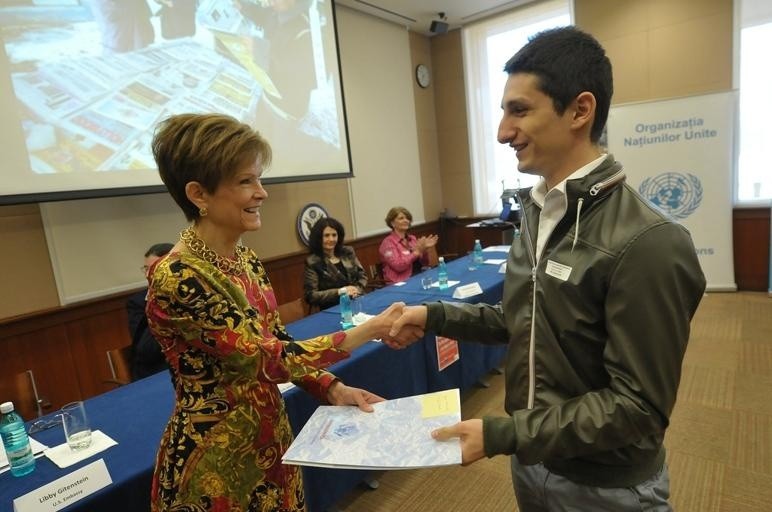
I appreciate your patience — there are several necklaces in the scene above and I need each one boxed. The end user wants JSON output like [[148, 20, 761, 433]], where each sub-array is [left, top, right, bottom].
[[178, 227, 248, 276]]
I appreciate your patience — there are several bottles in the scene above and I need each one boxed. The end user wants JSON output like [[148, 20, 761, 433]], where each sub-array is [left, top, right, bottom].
[[337, 287, 354, 330], [473, 239, 483, 264], [438, 256, 449, 291], [0, 401, 37, 477]]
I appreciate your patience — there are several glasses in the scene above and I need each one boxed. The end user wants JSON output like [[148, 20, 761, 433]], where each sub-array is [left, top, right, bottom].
[[28, 412, 70, 436]]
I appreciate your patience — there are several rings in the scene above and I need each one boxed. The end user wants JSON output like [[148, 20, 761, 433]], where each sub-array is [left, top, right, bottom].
[[430, 239, 433, 240]]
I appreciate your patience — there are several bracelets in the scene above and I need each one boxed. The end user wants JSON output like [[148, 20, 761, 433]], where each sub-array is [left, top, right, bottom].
[[339, 288, 346, 295]]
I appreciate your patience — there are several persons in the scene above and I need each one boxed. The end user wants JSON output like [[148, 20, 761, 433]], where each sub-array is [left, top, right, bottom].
[[143, 110, 428, 511], [83, 0, 321, 117], [303, 217, 370, 312], [124, 242, 174, 383], [376, 22, 709, 510], [377, 206, 440, 286]]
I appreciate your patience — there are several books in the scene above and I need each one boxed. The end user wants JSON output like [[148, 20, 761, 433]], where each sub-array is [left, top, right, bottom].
[[281, 387, 464, 471]]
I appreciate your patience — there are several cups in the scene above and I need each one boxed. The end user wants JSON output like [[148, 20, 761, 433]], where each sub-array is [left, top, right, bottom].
[[421, 266, 433, 290], [60, 400, 92, 453], [352, 294, 364, 314], [467, 251, 477, 270]]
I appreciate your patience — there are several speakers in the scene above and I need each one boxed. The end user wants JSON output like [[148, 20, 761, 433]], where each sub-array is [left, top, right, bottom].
[[431, 21, 450, 34]]
